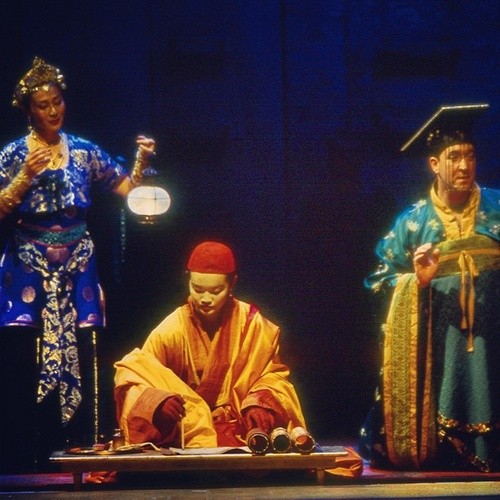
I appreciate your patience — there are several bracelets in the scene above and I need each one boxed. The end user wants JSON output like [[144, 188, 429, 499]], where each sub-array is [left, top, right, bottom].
[[0, 170, 34, 217], [128, 151, 150, 190]]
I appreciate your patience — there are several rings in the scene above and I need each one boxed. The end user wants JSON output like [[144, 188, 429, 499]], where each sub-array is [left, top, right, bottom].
[[38, 160, 41, 164]]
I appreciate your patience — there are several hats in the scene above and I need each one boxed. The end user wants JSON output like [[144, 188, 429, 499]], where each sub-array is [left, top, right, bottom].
[[187, 242, 237, 274], [400, 103, 490, 193], [12, 56, 66, 114]]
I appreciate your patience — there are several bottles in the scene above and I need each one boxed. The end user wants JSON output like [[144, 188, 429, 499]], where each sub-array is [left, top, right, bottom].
[[113, 429, 125, 452]]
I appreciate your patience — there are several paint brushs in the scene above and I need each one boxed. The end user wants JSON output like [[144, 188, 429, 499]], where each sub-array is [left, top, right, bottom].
[[181, 415, 185, 451]]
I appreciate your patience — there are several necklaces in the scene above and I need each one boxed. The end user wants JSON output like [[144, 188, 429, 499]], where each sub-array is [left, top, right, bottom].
[[31, 127, 61, 167]]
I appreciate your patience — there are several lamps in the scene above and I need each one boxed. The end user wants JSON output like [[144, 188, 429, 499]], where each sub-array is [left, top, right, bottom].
[[127, 140, 172, 223]]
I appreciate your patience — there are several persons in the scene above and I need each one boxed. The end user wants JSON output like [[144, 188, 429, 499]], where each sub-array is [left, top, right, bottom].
[[85, 241, 363, 484], [0, 56, 156, 472], [363, 104, 500, 473]]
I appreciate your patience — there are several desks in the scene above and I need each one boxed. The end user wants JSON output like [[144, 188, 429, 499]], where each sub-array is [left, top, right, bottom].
[[48, 445, 349, 489]]
